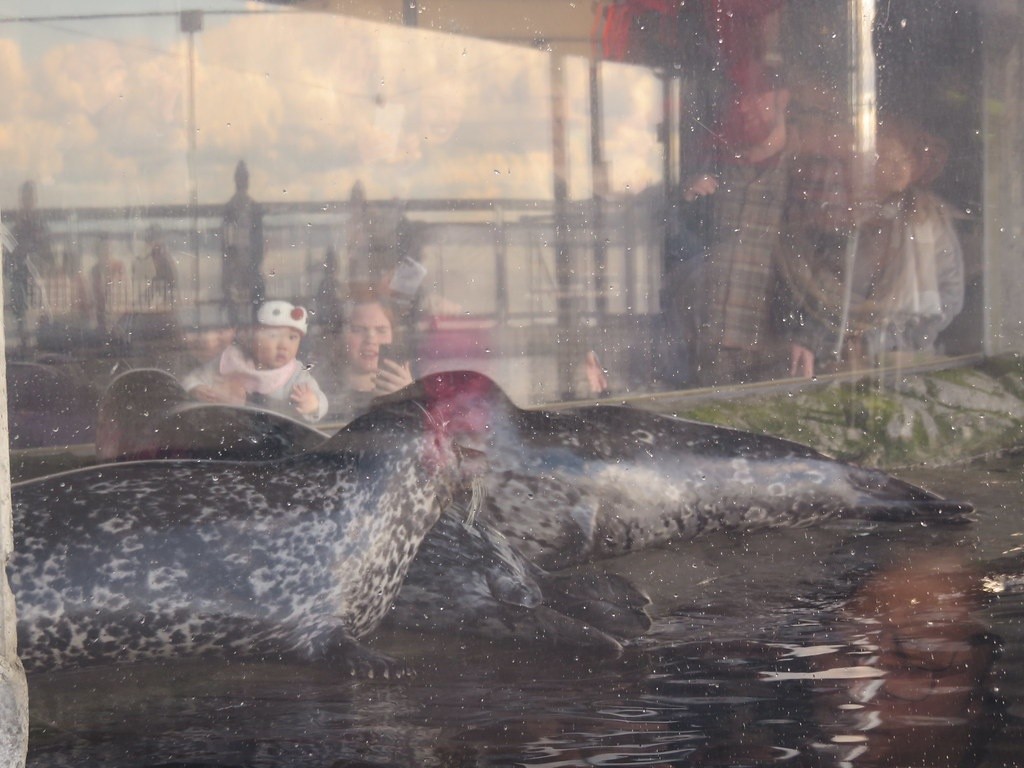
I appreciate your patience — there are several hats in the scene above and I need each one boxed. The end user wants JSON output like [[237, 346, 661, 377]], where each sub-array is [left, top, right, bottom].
[[234, 293, 308, 336]]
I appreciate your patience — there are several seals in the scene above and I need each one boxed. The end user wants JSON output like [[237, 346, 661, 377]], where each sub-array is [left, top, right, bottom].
[[102, 407, 543, 629], [532, 406, 976, 558], [4, 371, 581, 671]]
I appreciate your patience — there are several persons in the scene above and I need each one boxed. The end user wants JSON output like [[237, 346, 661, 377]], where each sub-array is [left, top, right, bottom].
[[672, 59, 965, 385], [180, 297, 328, 427], [8, 176, 431, 341], [303, 285, 415, 422]]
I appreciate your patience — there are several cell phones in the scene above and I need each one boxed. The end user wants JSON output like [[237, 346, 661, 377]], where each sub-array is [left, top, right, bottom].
[[377, 344, 407, 371]]
